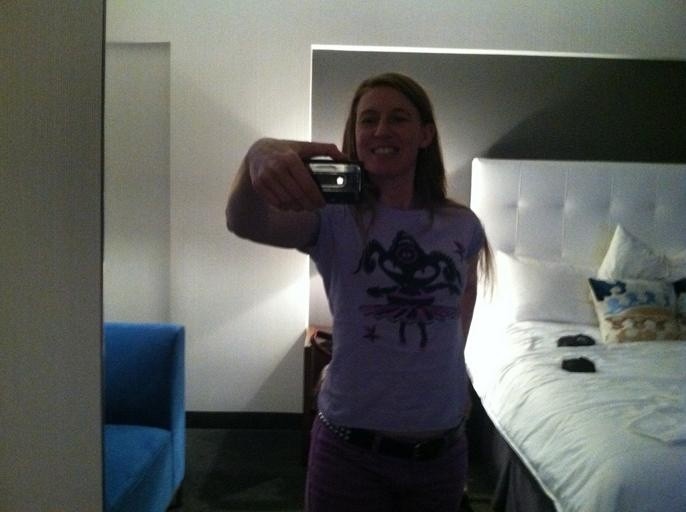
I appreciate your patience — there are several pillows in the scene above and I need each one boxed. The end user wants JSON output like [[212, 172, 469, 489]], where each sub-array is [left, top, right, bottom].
[[498, 251, 598, 328], [589, 222, 686, 299], [604, 306, 685, 342], [590, 279, 685, 321]]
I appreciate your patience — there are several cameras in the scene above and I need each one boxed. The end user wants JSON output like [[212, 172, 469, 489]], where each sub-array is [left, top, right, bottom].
[[306, 158, 365, 204]]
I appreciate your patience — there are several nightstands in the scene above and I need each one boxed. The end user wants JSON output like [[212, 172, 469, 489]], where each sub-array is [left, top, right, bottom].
[[303, 323, 336, 421]]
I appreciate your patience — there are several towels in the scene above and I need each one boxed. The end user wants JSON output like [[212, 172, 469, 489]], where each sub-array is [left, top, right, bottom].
[[627, 407, 685, 442]]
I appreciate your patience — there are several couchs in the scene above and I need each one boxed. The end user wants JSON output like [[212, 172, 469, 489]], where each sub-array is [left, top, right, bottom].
[[102, 324, 187, 507]]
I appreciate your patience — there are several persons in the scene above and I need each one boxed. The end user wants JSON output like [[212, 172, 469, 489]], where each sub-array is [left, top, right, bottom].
[[225, 72, 499, 511]]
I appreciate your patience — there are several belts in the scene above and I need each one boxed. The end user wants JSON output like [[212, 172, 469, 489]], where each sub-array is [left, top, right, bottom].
[[317, 406, 469, 462]]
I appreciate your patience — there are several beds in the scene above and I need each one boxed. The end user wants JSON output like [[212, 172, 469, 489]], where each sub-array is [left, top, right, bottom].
[[463, 158, 685, 507]]
